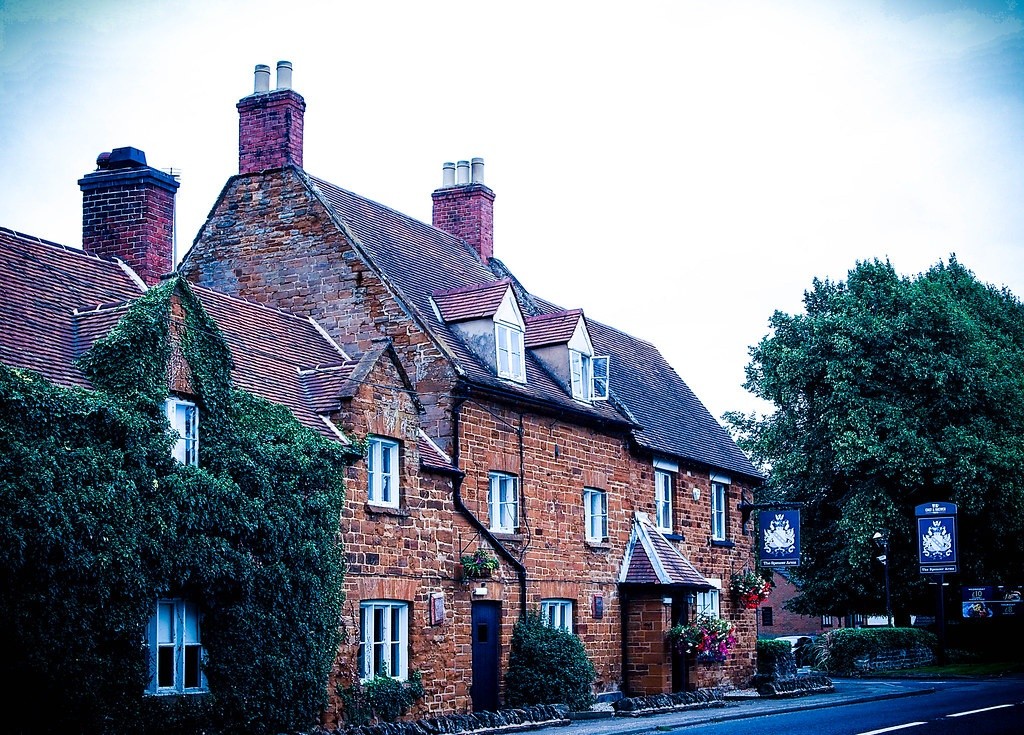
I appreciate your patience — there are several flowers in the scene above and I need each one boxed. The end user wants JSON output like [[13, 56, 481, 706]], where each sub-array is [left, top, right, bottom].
[[462, 548, 500, 577], [729, 569, 772, 609], [665, 615, 737, 663]]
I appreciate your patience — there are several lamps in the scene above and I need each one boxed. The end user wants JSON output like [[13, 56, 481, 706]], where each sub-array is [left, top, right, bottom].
[[687, 592, 696, 606]]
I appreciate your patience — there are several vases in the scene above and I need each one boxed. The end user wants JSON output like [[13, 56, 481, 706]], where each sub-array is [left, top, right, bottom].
[[672, 642, 688, 655], [468, 568, 492, 578]]
